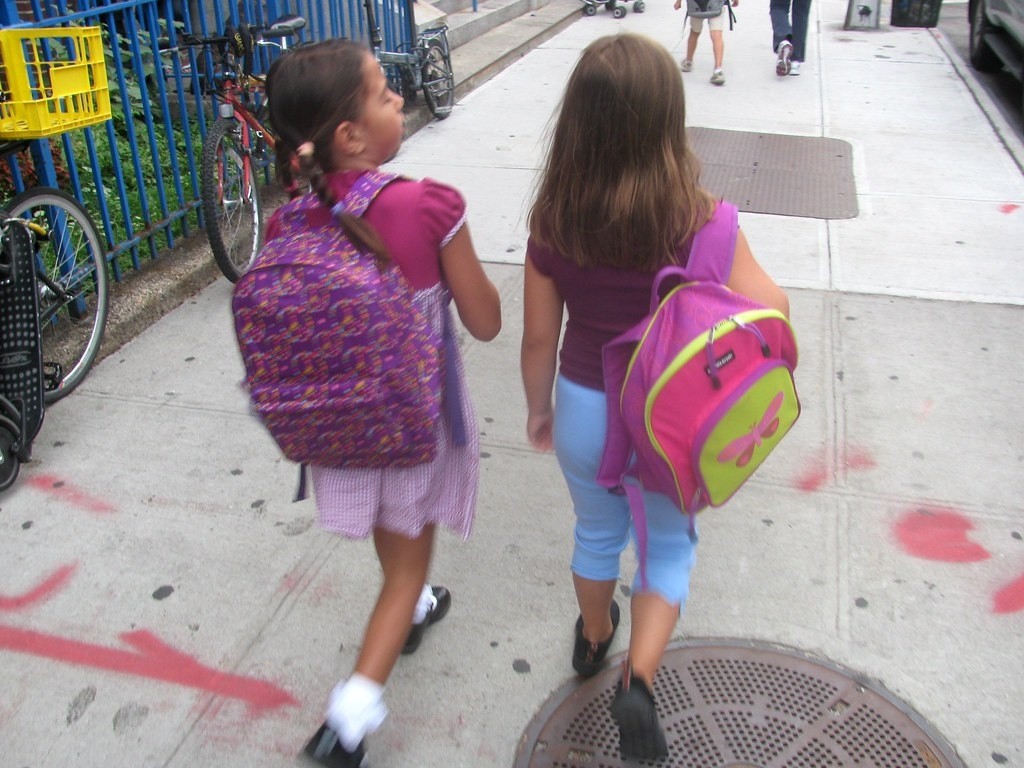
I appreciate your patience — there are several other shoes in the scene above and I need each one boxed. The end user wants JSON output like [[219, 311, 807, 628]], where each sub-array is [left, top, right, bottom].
[[572, 597, 620, 676], [402, 585, 451, 656], [295, 712, 368, 768], [608, 658, 670, 759]]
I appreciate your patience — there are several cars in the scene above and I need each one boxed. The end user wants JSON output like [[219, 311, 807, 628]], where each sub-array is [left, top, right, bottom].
[[965, 0, 1024, 90]]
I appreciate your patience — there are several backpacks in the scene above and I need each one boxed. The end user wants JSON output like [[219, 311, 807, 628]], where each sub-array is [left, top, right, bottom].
[[596, 199, 803, 588], [681, 0, 737, 31], [231, 169, 467, 503]]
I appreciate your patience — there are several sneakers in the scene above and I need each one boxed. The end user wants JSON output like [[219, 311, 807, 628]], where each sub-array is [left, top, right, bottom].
[[775, 39, 794, 77], [788, 59, 803, 75], [711, 71, 727, 85], [681, 59, 693, 73]]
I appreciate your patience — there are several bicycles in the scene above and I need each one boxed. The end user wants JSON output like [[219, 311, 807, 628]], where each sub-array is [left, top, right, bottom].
[[150, 0, 454, 283], [0, 139, 109, 488]]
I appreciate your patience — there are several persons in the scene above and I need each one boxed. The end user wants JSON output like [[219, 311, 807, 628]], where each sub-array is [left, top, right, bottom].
[[770, 0, 813, 76], [674, 0, 739, 83], [263, 38, 501, 768], [520, 35, 789, 757]]
